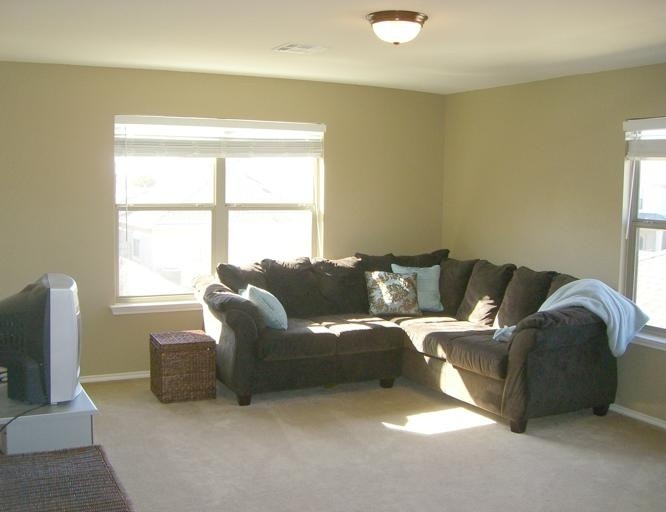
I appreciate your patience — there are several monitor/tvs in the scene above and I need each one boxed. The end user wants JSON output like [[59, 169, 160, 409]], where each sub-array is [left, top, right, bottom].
[[0, 272, 82, 406]]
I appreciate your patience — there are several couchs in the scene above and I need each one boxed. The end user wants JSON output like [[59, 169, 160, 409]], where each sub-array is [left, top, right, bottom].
[[195, 249, 618, 433]]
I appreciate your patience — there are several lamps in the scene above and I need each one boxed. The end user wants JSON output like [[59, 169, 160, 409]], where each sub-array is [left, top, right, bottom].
[[365, 11, 430, 46]]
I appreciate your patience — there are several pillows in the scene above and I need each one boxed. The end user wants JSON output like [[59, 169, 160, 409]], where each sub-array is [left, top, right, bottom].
[[234, 282, 291, 335], [361, 263, 446, 320]]
